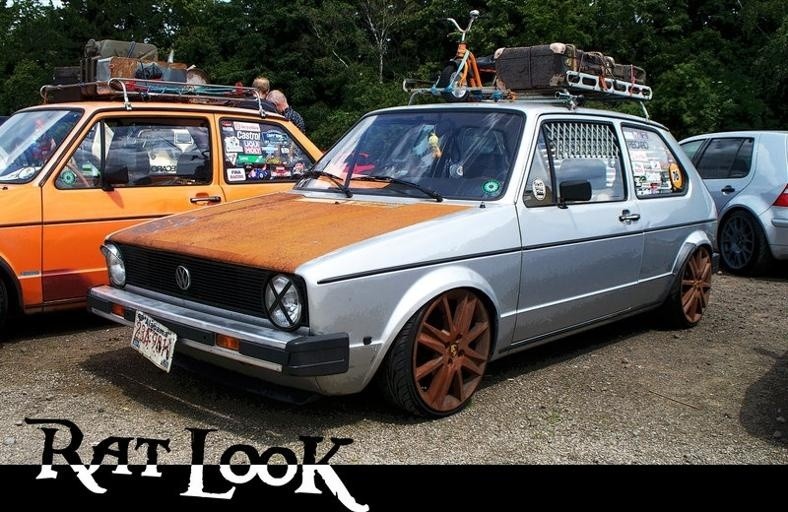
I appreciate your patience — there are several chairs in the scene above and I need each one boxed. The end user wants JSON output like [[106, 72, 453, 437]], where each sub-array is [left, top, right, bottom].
[[476, 153, 607, 191], [104, 143, 210, 184]]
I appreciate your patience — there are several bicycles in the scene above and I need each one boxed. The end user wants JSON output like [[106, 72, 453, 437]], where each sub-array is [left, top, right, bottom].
[[436, 10, 490, 102]]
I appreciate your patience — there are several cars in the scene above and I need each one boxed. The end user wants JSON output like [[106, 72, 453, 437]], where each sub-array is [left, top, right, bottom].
[[0, 70, 787, 420]]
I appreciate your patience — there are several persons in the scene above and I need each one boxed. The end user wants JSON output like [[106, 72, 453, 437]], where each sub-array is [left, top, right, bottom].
[[238, 75, 277, 112], [267, 90, 306, 133]]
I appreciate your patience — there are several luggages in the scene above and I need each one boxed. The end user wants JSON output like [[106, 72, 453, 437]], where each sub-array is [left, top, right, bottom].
[[493, 42, 649, 94], [47, 39, 187, 102]]
